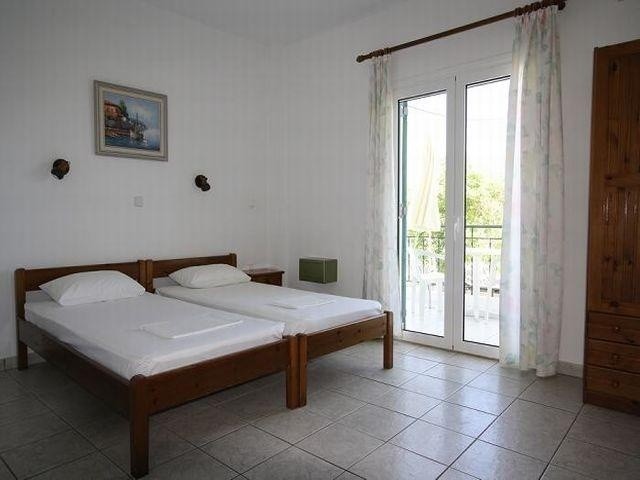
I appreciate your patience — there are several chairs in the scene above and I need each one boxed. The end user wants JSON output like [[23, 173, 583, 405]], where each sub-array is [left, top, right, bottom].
[[407, 245, 504, 323]]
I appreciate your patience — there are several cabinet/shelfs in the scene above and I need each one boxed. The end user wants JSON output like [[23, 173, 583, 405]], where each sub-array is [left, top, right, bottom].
[[580, 38, 640, 416]]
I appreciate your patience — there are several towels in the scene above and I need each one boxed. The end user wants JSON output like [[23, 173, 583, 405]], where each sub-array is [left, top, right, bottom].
[[137, 310, 244, 340], [265, 292, 337, 310]]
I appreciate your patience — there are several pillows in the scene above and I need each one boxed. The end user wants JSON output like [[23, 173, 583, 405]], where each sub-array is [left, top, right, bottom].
[[168, 263, 252, 289], [36, 269, 146, 306]]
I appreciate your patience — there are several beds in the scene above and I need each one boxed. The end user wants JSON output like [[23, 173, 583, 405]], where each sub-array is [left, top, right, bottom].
[[14, 259, 300, 480], [144, 252, 394, 408]]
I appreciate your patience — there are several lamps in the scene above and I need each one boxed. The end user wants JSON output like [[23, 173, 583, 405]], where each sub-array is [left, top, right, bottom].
[[195, 174, 210, 191], [50, 159, 70, 180]]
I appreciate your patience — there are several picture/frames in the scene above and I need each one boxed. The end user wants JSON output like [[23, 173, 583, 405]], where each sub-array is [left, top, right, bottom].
[[93, 79, 169, 162]]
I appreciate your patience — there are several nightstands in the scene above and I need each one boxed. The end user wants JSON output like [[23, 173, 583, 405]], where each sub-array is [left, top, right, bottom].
[[241, 267, 284, 286]]
